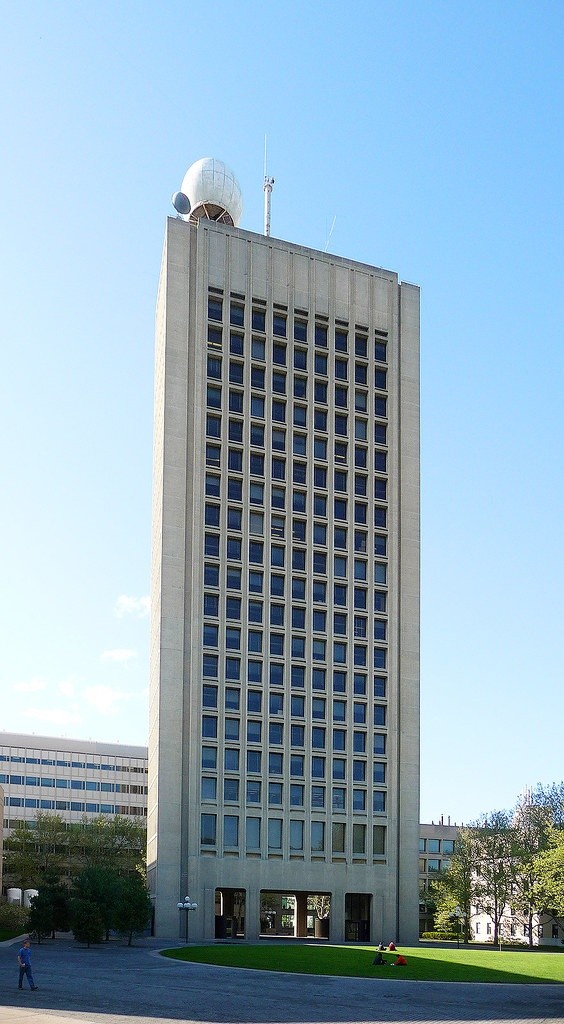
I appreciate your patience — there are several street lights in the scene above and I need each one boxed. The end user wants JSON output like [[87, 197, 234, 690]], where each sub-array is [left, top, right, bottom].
[[176, 896, 198, 943], [449, 906, 466, 949]]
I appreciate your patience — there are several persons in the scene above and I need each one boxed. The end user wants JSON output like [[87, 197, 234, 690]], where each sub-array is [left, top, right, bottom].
[[378, 941, 386, 951], [389, 941, 397, 951], [393, 954, 407, 965], [372, 953, 387, 965], [17, 940, 38, 991]]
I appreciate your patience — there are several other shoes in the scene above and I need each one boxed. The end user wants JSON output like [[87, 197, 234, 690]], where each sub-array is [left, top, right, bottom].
[[17, 987, 24, 990], [31, 987, 38, 991]]
